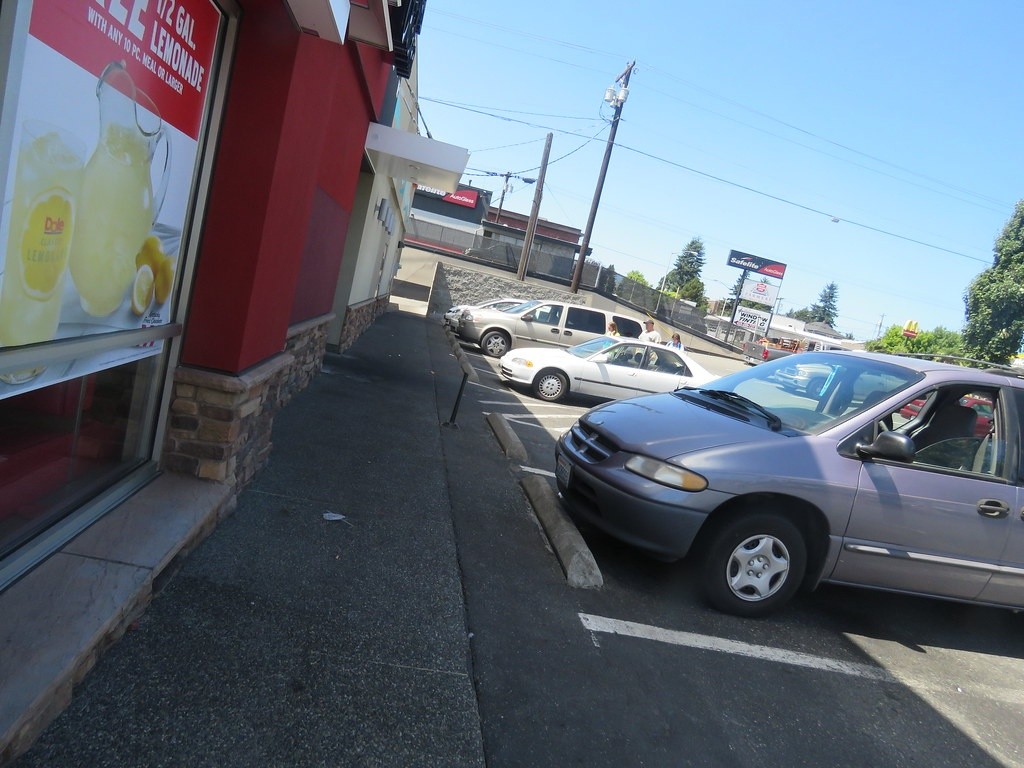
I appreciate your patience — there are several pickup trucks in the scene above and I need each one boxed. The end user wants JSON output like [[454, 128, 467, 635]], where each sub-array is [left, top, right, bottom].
[[744, 342, 793, 367]]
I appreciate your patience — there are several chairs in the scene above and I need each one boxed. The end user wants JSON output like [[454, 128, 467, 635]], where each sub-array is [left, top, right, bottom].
[[635, 353, 642, 361], [849, 391, 893, 445], [910, 406, 978, 469]]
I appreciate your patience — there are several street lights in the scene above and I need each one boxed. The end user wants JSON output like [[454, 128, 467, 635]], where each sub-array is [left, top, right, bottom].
[[712, 278, 732, 338], [654, 250, 698, 315]]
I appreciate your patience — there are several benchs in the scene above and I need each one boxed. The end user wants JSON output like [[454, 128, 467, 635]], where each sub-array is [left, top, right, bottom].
[[650, 359, 681, 375]]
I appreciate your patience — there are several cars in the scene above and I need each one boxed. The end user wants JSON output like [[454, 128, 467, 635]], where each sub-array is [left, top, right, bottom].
[[443, 299, 574, 333], [498, 335, 722, 402], [552, 350, 1024, 622], [775, 365, 886, 401]]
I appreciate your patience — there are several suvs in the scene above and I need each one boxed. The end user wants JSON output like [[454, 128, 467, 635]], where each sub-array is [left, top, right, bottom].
[[457, 298, 648, 360]]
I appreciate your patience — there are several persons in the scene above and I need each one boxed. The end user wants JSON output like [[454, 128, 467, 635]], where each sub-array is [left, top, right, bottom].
[[636, 320, 660, 364], [604, 321, 620, 352], [666, 333, 684, 351]]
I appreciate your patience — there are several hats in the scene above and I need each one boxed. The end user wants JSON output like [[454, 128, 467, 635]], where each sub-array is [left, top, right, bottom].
[[643, 320, 654, 325]]
